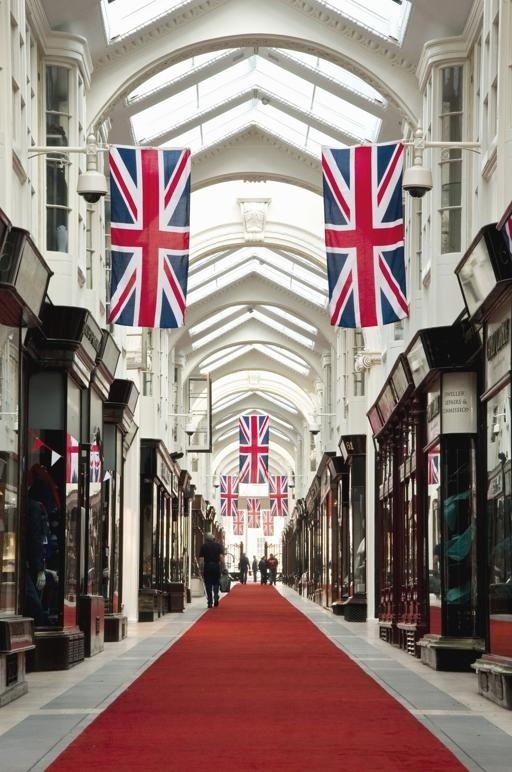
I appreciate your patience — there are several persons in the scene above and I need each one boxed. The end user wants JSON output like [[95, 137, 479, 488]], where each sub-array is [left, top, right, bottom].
[[201, 532, 225, 607], [239, 553, 251, 585], [259, 556, 271, 586], [20, 492, 59, 618], [268, 553, 278, 585], [252, 555, 258, 581]]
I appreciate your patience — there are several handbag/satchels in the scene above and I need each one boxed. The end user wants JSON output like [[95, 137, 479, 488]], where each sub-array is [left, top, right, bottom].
[[219, 575, 230, 592]]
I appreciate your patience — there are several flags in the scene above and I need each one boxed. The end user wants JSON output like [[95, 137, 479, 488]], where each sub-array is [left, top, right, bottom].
[[268, 475, 289, 516], [426, 447, 440, 485], [90, 442, 102, 482], [320, 141, 409, 328], [105, 145, 192, 330], [219, 475, 239, 517], [233, 510, 244, 534], [238, 415, 271, 482], [246, 498, 260, 527], [263, 510, 274, 536], [65, 435, 79, 483]]
